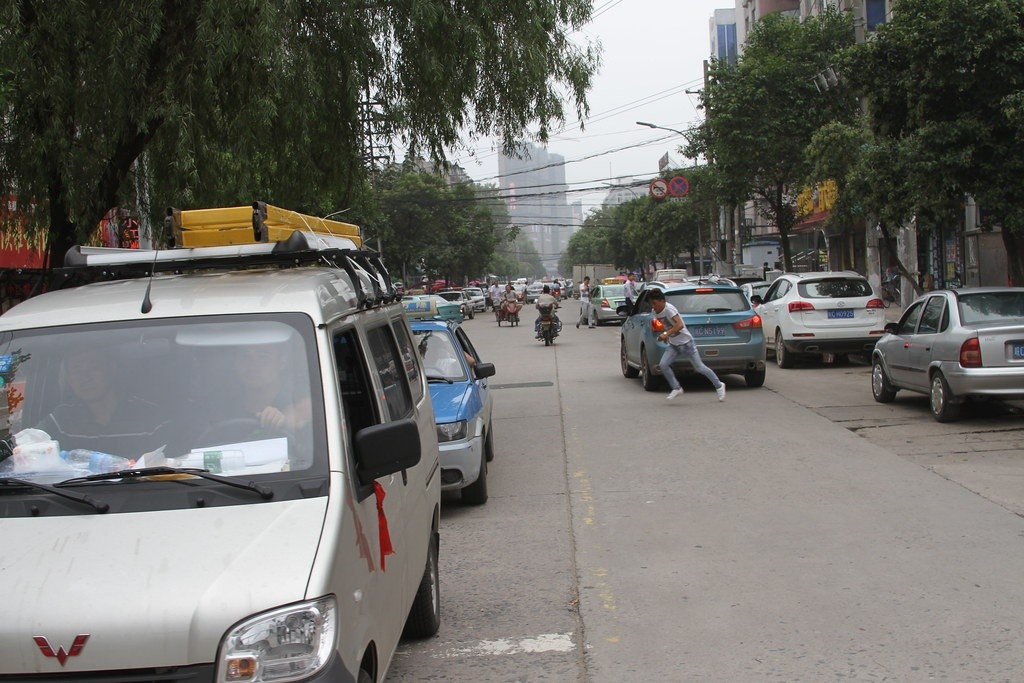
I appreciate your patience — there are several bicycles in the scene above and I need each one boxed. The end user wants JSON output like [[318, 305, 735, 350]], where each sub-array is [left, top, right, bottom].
[[877, 275, 901, 309]]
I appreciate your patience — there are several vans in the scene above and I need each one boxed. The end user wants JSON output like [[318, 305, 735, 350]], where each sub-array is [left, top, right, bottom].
[[0, 231, 443, 683]]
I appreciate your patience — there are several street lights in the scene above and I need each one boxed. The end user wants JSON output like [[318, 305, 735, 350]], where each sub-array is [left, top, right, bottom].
[[635, 121, 703, 278], [603, 183, 651, 284]]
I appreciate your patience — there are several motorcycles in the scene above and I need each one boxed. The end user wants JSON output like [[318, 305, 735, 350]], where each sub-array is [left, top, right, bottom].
[[535, 298, 562, 346]]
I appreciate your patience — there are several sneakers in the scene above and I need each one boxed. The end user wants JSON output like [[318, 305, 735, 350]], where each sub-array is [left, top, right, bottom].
[[716, 383, 726, 401], [667, 388, 683, 400]]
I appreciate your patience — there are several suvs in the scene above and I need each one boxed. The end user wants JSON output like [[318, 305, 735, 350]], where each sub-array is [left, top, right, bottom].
[[616, 278, 766, 391], [750, 270, 887, 369]]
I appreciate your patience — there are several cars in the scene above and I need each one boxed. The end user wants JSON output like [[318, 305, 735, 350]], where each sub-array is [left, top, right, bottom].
[[580, 269, 773, 326], [396, 289, 463, 325], [400, 300, 496, 507], [394, 276, 573, 320], [872, 286, 1024, 422]]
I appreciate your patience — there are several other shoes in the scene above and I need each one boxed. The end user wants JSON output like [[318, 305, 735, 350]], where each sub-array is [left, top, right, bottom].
[[589, 327, 594, 329], [576, 323, 579, 328]]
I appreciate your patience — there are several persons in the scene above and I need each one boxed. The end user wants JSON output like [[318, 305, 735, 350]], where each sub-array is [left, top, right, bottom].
[[464, 351, 475, 365], [575, 276, 595, 328], [624, 273, 635, 305], [552, 279, 560, 290], [534, 286, 557, 339], [38, 353, 162, 443], [647, 288, 726, 401], [489, 281, 523, 312], [763, 262, 770, 280], [242, 348, 312, 432]]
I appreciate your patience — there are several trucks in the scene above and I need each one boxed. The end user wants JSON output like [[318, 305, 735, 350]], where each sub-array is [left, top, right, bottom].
[[573, 264, 629, 299]]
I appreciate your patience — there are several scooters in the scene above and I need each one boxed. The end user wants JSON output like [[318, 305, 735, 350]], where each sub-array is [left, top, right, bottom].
[[497, 298, 518, 326]]
